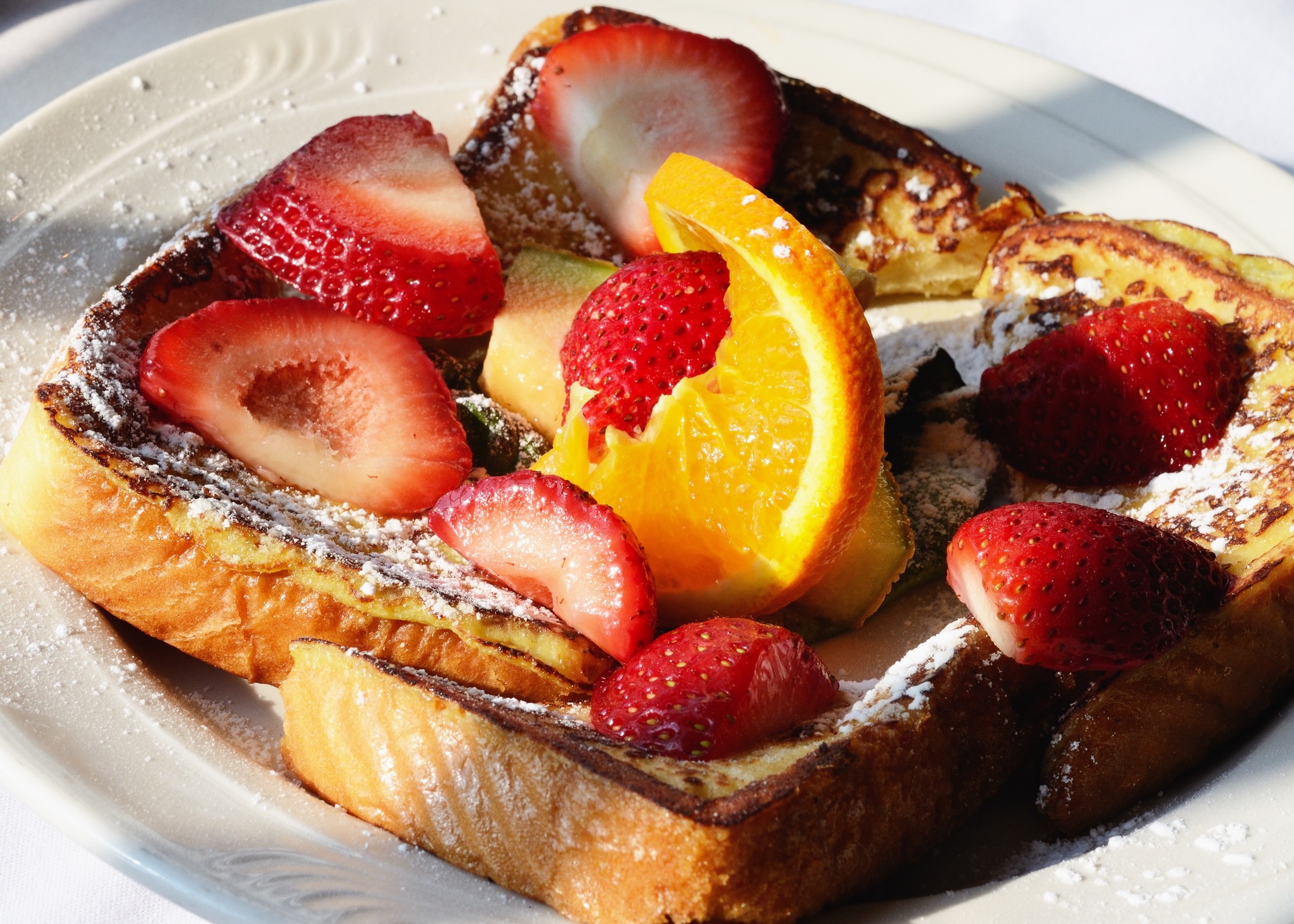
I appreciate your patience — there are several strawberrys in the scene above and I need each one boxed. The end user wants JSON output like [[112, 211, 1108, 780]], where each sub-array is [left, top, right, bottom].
[[153, 0, 1254, 767]]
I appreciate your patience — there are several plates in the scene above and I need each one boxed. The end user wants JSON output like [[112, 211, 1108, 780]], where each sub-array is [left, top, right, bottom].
[[1, 1, 1293, 924]]
